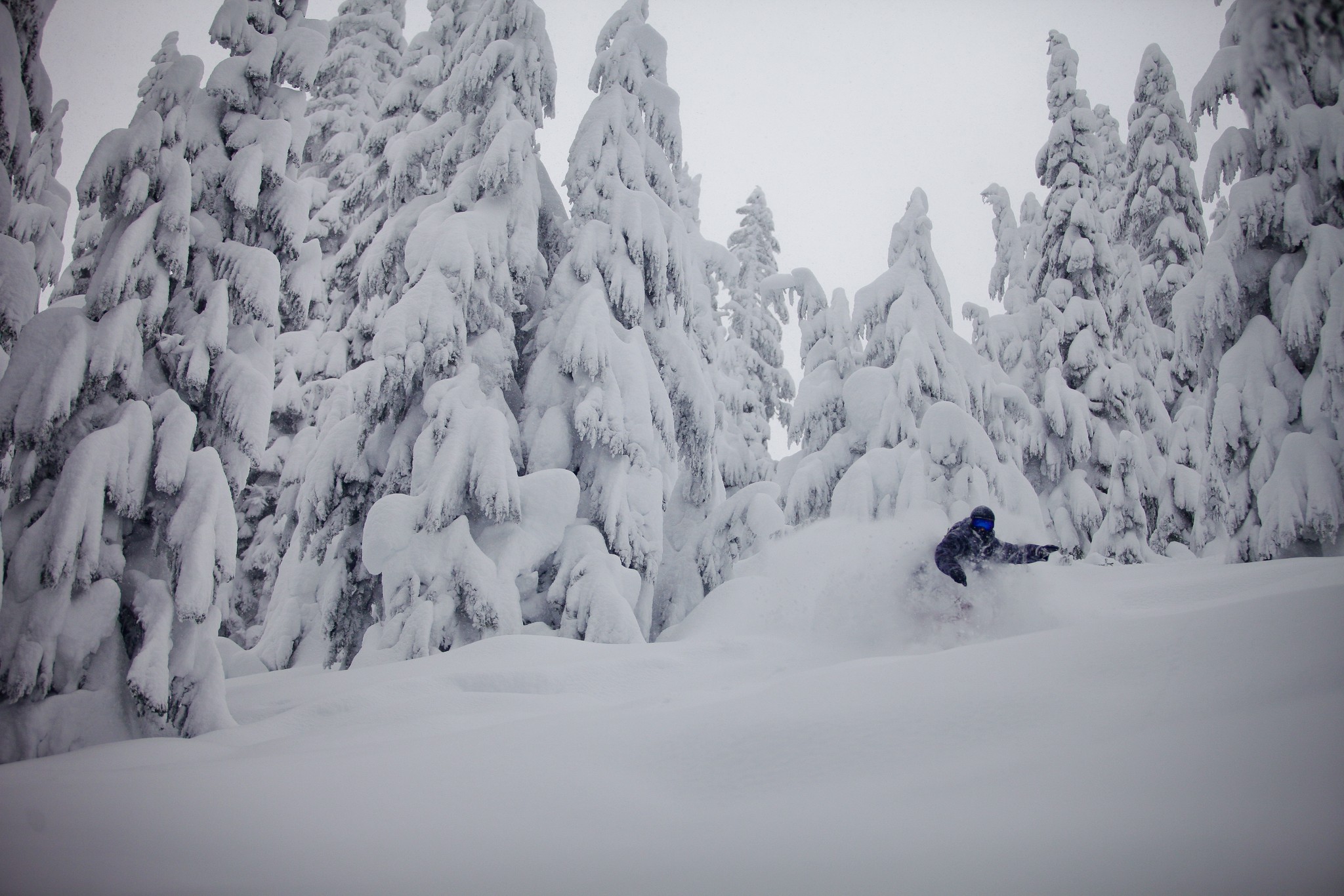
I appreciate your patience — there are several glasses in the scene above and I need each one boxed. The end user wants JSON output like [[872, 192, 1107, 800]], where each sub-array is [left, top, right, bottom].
[[970, 517, 995, 530]]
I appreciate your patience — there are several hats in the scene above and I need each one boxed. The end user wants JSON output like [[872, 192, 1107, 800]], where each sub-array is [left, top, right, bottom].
[[970, 505, 995, 521]]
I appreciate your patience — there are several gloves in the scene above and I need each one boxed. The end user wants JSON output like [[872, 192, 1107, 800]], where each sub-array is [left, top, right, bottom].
[[1037, 544, 1059, 560], [949, 567, 967, 587]]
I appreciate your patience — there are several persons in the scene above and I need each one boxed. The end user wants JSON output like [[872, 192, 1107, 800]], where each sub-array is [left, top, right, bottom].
[[934, 508, 1060, 588]]
